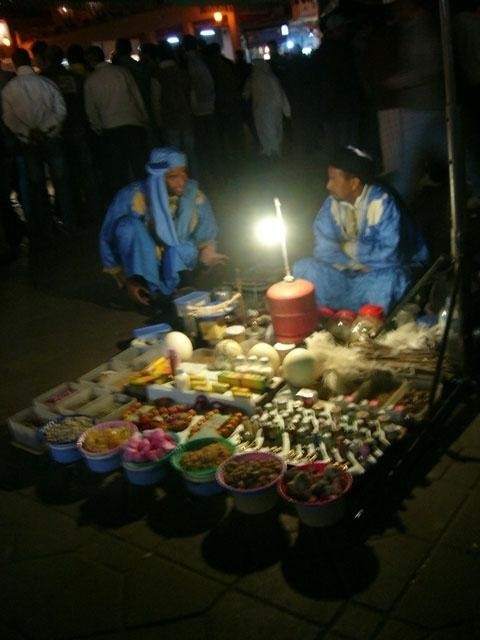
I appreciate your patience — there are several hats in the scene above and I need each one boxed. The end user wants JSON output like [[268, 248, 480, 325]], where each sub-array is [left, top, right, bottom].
[[328, 144, 376, 185]]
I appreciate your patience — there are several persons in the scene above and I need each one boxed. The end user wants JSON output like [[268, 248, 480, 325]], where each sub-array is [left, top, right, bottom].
[[97, 147, 233, 323], [309, 147, 431, 311], [3, 35, 300, 211], [375, 2, 447, 177]]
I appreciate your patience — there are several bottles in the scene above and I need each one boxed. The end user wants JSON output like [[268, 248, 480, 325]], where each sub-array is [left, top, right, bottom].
[[439, 296, 459, 332], [225, 325, 245, 343], [169, 348, 182, 377]]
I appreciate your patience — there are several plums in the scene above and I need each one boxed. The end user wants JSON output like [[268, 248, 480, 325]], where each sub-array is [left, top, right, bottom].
[[120, 402, 244, 438]]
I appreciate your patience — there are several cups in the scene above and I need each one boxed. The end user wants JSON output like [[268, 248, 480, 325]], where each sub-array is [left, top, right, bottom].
[[274, 341, 296, 360], [214, 286, 231, 301]]
